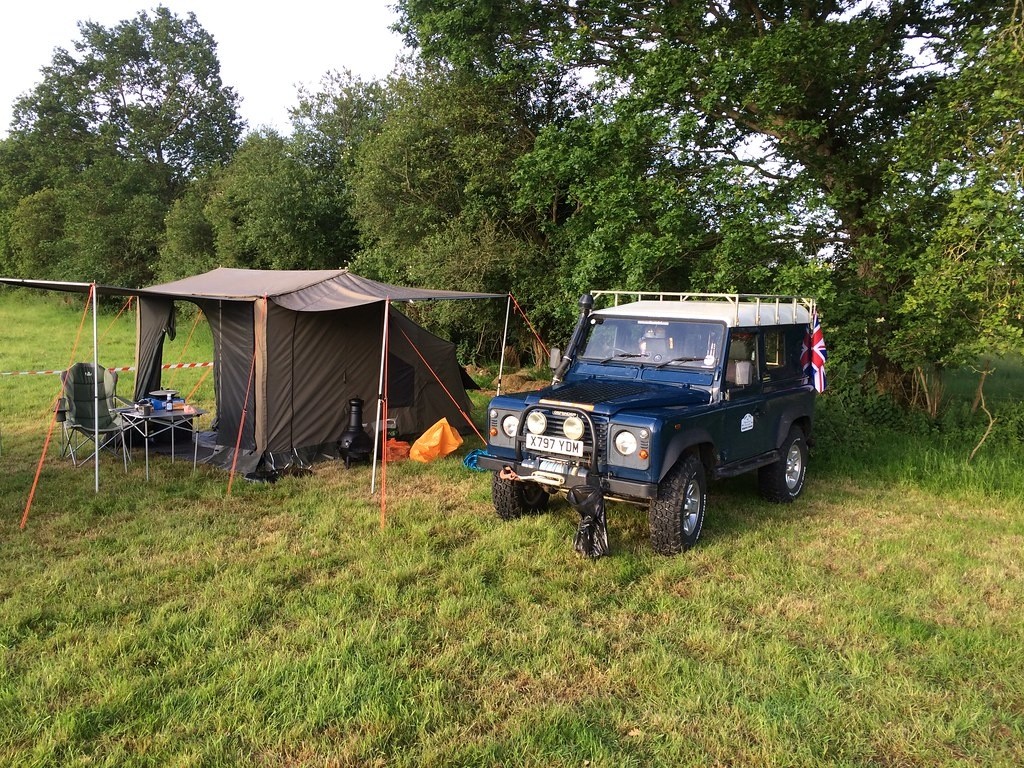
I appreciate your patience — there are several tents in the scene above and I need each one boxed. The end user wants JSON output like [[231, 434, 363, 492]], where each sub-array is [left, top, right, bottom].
[[0, 267, 507, 476]]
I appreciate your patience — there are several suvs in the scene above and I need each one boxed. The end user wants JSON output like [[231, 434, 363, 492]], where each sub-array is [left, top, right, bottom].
[[477, 290, 818, 557]]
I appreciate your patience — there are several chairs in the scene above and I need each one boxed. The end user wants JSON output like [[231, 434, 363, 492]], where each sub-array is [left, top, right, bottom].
[[640, 327, 668, 356], [54, 363, 132, 469], [724, 340, 753, 386]]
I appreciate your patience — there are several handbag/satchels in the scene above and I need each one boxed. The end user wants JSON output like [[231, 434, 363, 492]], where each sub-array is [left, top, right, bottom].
[[386, 437, 412, 463]]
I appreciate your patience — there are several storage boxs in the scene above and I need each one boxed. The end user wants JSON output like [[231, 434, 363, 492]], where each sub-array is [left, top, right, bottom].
[[152, 414, 193, 443]]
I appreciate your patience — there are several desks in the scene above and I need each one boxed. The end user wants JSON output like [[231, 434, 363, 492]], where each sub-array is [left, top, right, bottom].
[[115, 407, 211, 480]]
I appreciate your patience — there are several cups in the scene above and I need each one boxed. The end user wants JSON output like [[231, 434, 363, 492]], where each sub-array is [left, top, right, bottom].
[[138, 404, 150, 415]]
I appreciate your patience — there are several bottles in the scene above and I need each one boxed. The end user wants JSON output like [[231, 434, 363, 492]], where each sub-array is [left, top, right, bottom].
[[167, 394, 173, 411]]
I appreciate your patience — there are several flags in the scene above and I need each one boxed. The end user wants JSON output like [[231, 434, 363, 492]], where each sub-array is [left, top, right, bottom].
[[811, 304, 828, 394], [799, 323, 814, 392]]
